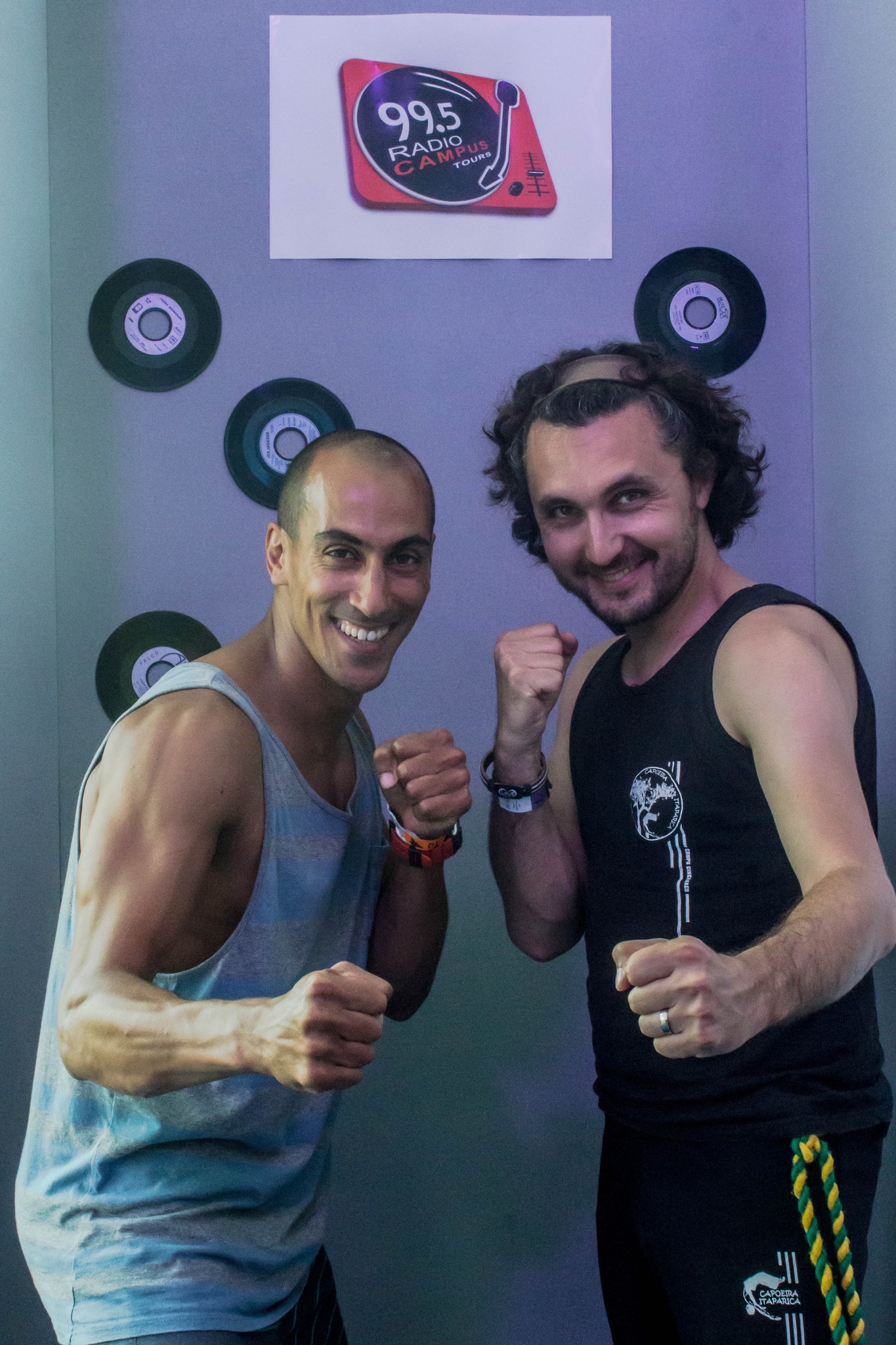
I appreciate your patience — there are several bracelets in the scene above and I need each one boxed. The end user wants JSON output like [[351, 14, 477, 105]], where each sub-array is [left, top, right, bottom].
[[478, 747, 553, 813], [386, 810, 463, 868]]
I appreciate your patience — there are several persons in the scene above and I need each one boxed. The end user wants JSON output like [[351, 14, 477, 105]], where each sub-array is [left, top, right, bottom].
[[12, 432, 473, 1345], [465, 339, 896, 1345]]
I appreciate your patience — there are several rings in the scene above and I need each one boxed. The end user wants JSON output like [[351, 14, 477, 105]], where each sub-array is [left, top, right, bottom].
[[659, 1008, 675, 1037]]
